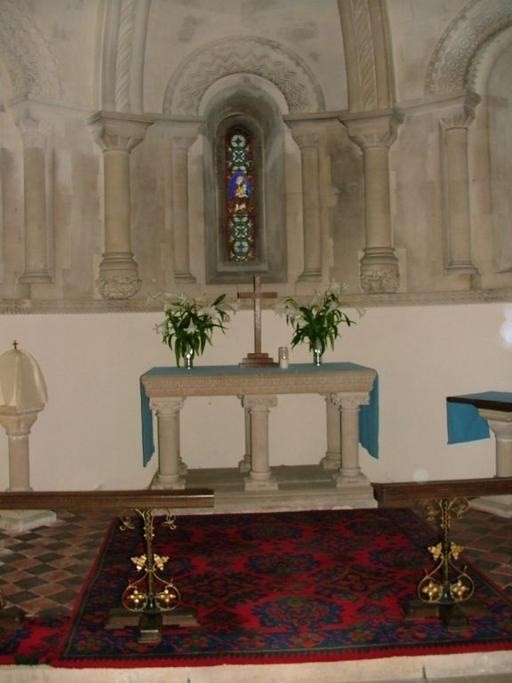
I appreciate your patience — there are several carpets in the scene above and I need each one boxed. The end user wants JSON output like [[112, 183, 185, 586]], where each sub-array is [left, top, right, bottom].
[[49, 508, 512, 669]]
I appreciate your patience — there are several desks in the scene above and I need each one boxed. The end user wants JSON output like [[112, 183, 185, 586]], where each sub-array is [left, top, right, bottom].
[[141, 362, 378, 514], [447, 391, 512, 478]]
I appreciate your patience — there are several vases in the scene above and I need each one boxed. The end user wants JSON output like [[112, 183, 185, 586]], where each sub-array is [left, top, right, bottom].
[[314, 344, 321, 366], [185, 348, 193, 369]]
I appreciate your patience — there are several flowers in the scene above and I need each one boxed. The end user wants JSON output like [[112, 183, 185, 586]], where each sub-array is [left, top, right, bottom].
[[154, 291, 240, 368], [279, 278, 365, 362]]
